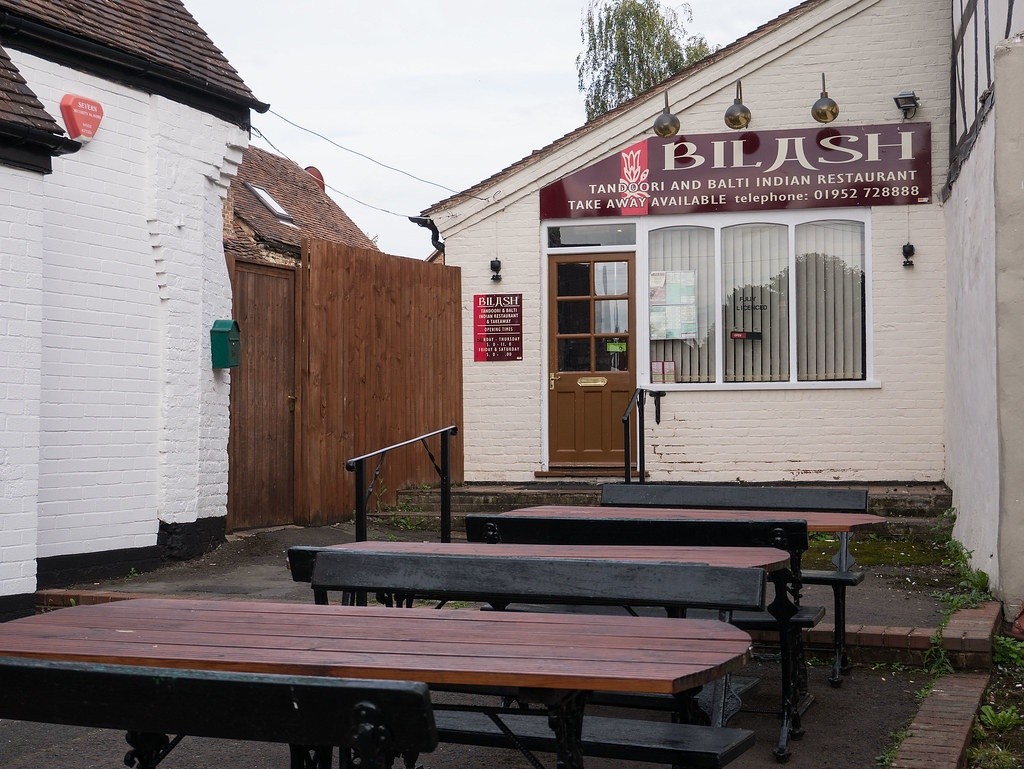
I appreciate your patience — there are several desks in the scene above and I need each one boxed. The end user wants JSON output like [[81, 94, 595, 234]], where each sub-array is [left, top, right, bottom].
[[495, 507, 887, 687], [286, 540, 807, 761], [0, 599, 752, 768]]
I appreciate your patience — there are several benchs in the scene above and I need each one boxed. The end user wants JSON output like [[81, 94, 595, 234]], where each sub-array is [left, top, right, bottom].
[[0, 485, 888, 769]]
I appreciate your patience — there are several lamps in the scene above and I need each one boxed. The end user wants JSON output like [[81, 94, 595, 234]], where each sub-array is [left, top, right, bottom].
[[724, 78, 751, 128], [811, 72, 839, 124], [893, 90, 921, 118], [652, 88, 681, 137]]
